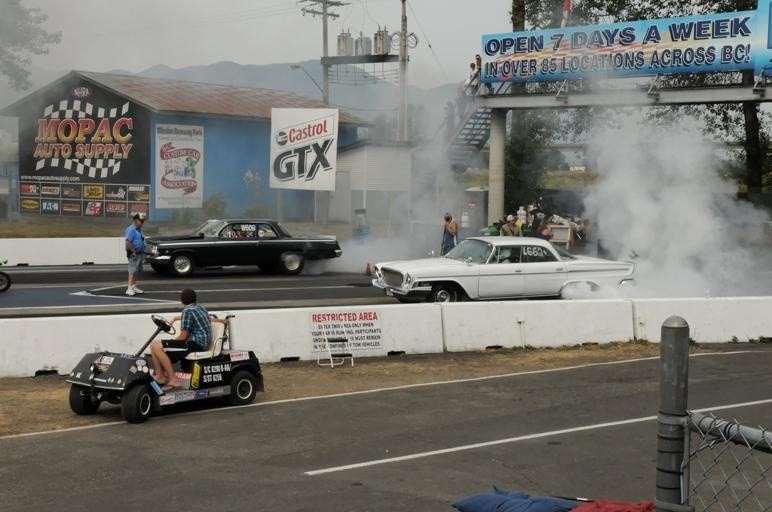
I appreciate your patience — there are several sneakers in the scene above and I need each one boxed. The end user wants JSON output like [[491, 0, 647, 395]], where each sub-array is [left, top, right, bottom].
[[133, 286, 145, 294], [126, 287, 135, 296]]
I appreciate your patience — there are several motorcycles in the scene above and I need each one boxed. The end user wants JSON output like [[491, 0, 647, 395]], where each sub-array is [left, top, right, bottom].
[[0, 272, 11, 292]]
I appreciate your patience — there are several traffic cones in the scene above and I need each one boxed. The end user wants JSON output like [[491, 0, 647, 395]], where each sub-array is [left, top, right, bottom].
[[366, 262, 372, 273]]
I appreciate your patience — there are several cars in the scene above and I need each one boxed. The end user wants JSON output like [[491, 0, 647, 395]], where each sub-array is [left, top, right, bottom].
[[371, 235, 632, 302], [144, 218, 342, 277]]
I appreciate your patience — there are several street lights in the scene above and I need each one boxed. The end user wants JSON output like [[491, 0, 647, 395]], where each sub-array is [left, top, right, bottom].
[[290, 61, 330, 104]]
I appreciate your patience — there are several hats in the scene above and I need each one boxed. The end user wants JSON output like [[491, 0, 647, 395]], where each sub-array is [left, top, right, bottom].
[[507, 214, 517, 221], [133, 213, 147, 221]]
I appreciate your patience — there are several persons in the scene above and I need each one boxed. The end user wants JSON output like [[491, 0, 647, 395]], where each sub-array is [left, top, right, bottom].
[[440, 212, 458, 257], [454, 88, 466, 118], [125, 213, 147, 296], [232, 227, 243, 240], [469, 63, 478, 93], [499, 215, 523, 237], [152, 288, 211, 393], [443, 100, 454, 131], [475, 55, 492, 92], [537, 223, 553, 241]]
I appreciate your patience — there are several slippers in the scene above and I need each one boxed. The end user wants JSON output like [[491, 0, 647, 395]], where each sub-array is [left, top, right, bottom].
[[163, 382, 181, 392]]
